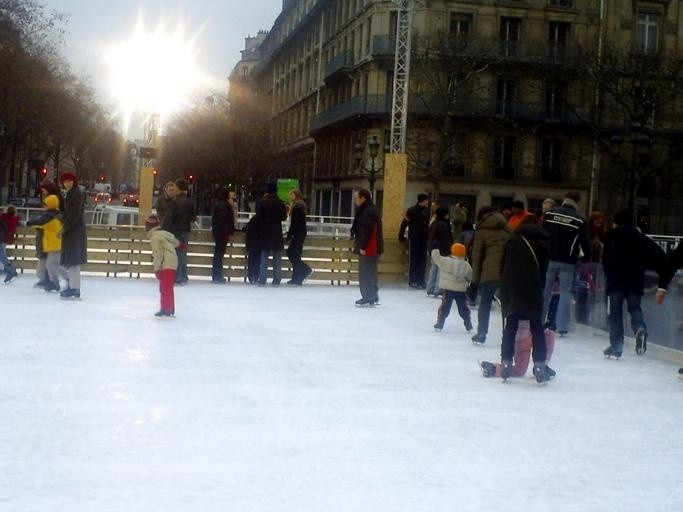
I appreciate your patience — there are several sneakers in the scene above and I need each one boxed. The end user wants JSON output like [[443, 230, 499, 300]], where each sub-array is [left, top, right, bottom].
[[4, 271, 79, 297], [534, 323, 646, 382], [155, 311, 170, 316], [356, 298, 374, 304], [406, 281, 513, 376], [176, 269, 311, 285]]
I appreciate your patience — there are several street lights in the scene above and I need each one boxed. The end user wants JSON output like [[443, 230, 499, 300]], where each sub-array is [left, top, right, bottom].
[[137, 113, 164, 226], [351, 133, 390, 205]]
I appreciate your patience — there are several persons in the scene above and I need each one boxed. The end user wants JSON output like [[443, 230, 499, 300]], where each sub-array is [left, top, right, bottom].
[[601, 208, 666, 359], [656, 238, 682, 373], [500, 216, 555, 380], [0, 173, 88, 300], [349, 187, 384, 307], [478, 274, 558, 378], [212, 182, 313, 286], [145, 178, 197, 320], [398, 190, 606, 344]]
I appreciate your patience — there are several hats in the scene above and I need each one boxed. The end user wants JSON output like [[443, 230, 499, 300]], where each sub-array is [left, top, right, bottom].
[[60, 173, 75, 184], [450, 243, 466, 256], [613, 210, 632, 225], [436, 207, 448, 216], [512, 201, 523, 209], [417, 193, 427, 202], [146, 216, 160, 227]]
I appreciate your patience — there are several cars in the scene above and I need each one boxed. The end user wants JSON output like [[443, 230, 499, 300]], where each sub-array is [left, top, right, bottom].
[[51, 179, 139, 208]]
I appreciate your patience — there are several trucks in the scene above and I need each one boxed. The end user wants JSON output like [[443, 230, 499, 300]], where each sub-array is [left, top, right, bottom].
[[88, 205, 159, 226]]
